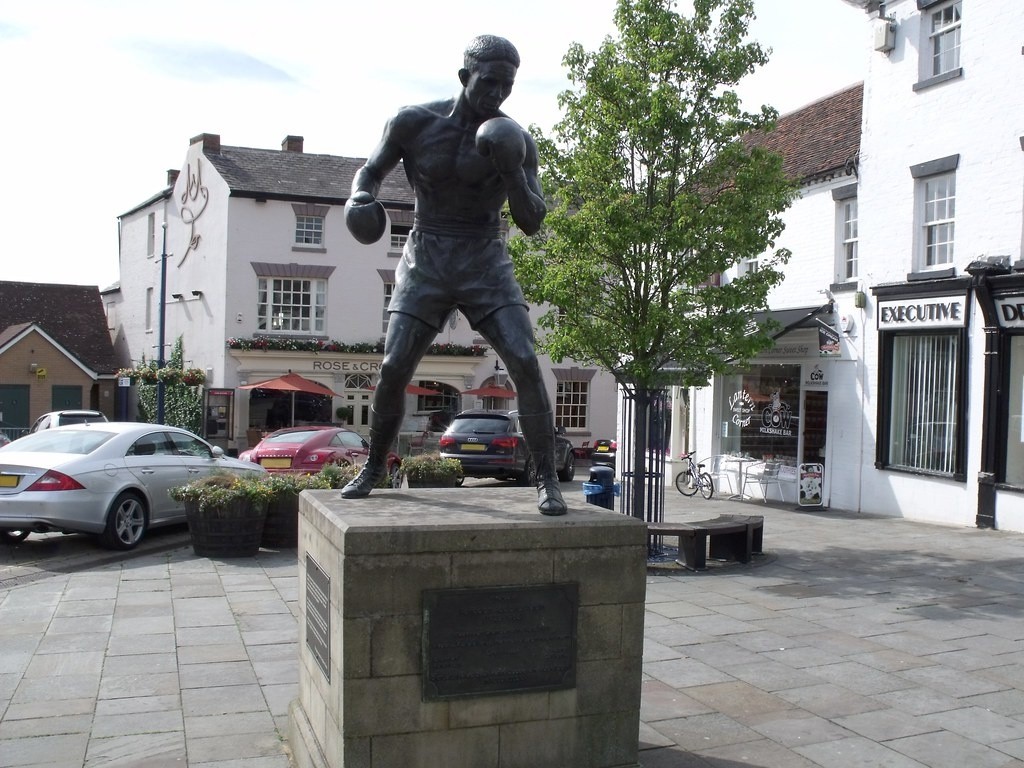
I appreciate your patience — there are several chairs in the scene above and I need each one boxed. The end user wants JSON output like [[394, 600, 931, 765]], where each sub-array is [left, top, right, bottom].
[[696, 454, 734, 499], [741, 459, 785, 503]]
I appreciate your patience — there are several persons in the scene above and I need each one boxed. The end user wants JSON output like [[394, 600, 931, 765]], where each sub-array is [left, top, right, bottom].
[[340, 34, 567, 515]]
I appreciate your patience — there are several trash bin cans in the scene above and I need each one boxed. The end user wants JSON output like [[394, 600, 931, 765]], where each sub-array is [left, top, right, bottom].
[[582, 466, 614, 511]]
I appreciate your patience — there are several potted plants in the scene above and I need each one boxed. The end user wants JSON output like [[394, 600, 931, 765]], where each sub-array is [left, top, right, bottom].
[[169, 453, 464, 559]]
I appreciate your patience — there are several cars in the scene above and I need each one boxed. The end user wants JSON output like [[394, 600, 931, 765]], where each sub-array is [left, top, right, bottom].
[[590, 438, 616, 478], [236, 425, 405, 490], [0, 432, 12, 450], [21, 410, 110, 436], [0, 422, 268, 550], [437, 408, 577, 487]]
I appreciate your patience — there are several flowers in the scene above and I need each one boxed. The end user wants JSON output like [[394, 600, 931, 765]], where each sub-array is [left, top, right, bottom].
[[183, 369, 206, 383], [115, 367, 140, 380], [157, 366, 183, 386], [137, 367, 157, 383]]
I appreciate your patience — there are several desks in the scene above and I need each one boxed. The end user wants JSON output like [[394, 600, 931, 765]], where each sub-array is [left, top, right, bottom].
[[725, 459, 757, 500]]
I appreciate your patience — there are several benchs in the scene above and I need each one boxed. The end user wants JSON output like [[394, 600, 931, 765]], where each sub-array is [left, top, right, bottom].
[[644, 513, 764, 572]]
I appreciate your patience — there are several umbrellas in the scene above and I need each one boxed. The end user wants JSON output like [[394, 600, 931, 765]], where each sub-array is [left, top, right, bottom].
[[236, 374, 344, 427], [363, 383, 444, 395], [456, 384, 518, 410]]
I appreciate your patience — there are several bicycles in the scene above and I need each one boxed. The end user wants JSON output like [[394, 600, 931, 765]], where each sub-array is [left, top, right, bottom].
[[675, 451, 714, 500]]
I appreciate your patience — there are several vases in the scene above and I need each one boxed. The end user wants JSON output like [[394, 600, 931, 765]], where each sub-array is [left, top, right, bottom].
[[130, 377, 137, 385], [162, 377, 175, 386], [186, 382, 200, 386], [145, 379, 157, 385]]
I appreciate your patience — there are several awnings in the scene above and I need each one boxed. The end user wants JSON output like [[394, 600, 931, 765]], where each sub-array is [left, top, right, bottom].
[[610, 303, 831, 386]]
